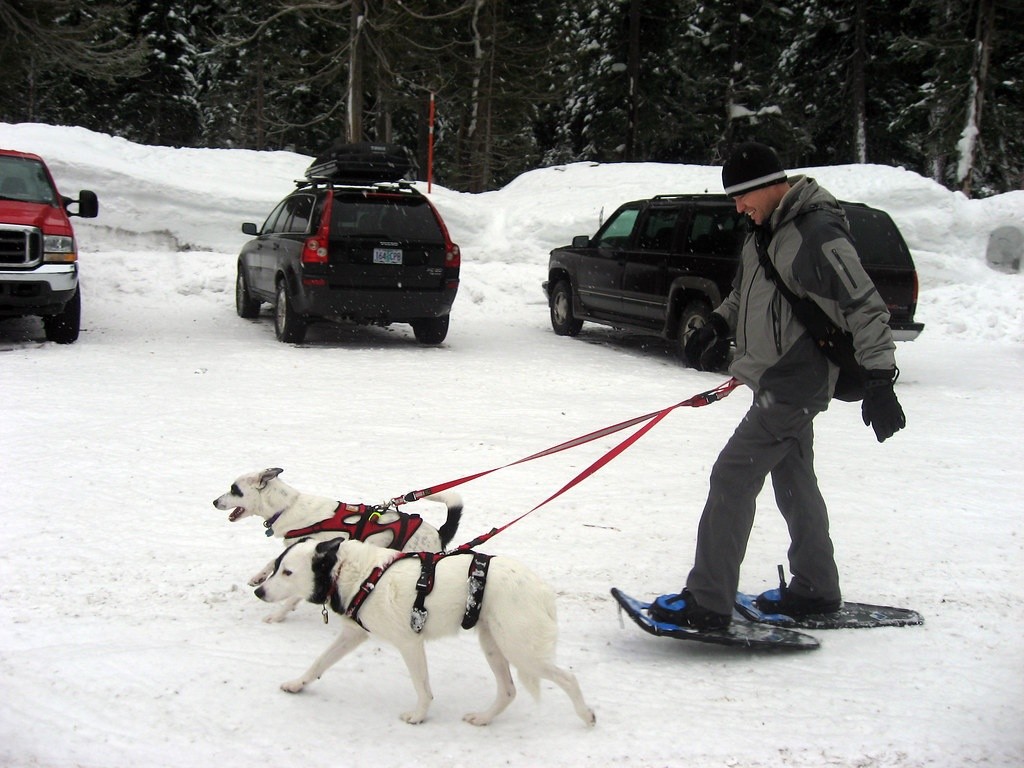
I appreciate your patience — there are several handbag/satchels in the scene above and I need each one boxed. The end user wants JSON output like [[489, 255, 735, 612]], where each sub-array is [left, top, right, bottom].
[[754, 234, 872, 403]]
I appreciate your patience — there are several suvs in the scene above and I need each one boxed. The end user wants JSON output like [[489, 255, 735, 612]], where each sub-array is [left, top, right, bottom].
[[235, 175, 461, 344], [0, 150, 99, 345], [540, 190, 926, 374]]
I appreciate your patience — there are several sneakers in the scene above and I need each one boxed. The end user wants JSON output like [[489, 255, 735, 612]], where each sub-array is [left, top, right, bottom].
[[648, 590, 731, 631], [757, 584, 839, 618]]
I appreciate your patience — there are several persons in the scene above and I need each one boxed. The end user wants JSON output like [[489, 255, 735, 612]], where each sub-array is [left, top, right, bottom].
[[648, 142, 907, 628]]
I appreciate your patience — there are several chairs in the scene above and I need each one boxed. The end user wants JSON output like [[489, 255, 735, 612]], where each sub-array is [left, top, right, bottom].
[[0, 177, 27, 193], [651, 227, 674, 251], [336, 206, 396, 232]]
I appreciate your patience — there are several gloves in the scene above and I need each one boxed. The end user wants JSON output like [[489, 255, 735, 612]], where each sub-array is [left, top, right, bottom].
[[862, 371, 907, 443], [685, 311, 729, 371]]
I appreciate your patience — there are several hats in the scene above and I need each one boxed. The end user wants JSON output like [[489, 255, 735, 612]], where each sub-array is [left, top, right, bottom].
[[722, 141, 787, 198]]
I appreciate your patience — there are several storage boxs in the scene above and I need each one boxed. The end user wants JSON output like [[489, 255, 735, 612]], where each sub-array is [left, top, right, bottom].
[[305, 140, 413, 182]]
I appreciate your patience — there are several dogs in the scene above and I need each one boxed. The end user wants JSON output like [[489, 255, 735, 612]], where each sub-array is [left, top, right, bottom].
[[212, 466, 599, 734]]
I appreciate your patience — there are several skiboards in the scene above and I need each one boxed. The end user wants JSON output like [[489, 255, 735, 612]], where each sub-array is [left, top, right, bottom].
[[611, 585, 925, 651]]
